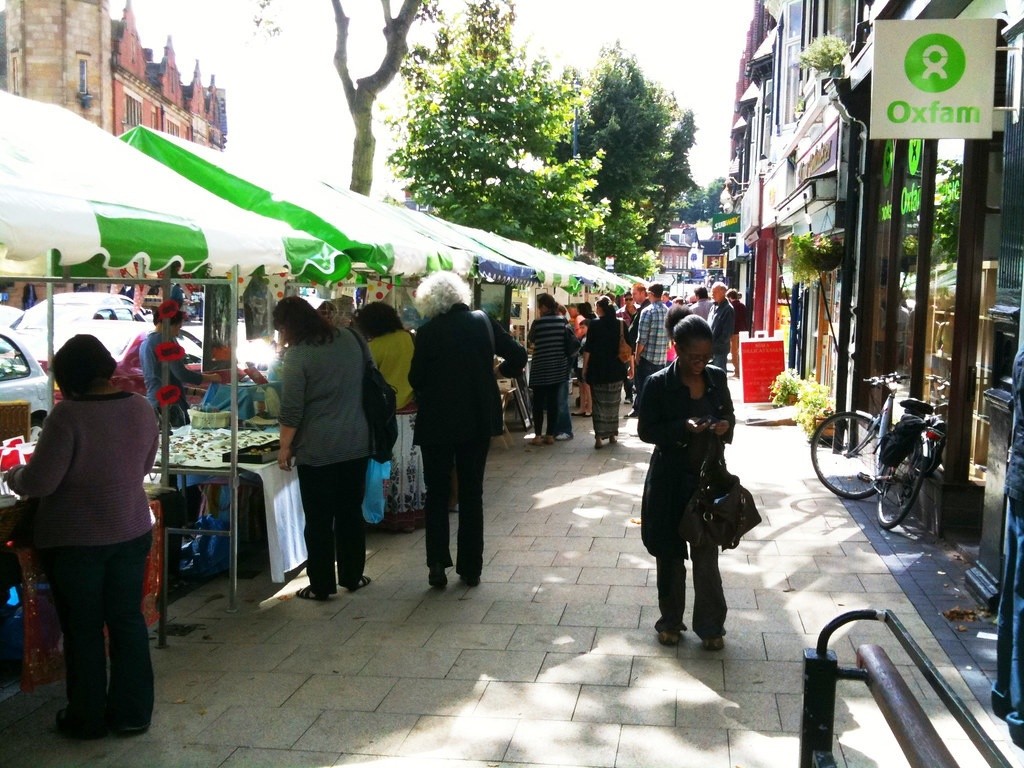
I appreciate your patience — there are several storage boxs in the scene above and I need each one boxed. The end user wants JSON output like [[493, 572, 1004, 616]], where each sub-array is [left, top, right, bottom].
[[222, 445, 281, 463], [187, 405, 231, 429]]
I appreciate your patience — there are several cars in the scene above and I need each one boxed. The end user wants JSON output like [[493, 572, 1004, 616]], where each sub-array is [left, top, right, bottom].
[[0, 292, 205, 446]]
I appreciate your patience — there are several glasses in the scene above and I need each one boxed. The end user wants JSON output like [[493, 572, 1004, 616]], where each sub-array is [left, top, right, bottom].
[[680, 348, 715, 364]]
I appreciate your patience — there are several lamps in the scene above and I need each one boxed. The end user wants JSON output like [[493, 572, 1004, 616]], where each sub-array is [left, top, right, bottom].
[[720, 177, 750, 204], [723, 194, 743, 214]]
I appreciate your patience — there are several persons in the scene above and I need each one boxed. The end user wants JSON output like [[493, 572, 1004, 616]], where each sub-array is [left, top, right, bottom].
[[636, 314, 736, 650], [878, 286, 955, 380], [2, 335, 159, 741], [139, 267, 752, 600], [989, 348, 1024, 750]]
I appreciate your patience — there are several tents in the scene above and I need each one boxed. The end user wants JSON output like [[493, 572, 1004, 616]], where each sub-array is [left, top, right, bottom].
[[0, 84, 652, 608]]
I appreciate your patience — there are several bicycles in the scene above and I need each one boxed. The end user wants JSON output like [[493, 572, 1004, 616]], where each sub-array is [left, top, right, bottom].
[[810, 372, 946, 530], [922, 373, 952, 474]]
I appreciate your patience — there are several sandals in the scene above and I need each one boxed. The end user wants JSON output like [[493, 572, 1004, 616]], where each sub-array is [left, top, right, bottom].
[[348, 576, 371, 589], [544, 437, 554, 444], [529, 437, 542, 445], [297, 586, 328, 599]]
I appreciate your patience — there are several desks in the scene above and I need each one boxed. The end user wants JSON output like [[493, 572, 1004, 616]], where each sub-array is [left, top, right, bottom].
[[148, 418, 302, 584], [0, 483, 174, 649], [202, 379, 283, 416]]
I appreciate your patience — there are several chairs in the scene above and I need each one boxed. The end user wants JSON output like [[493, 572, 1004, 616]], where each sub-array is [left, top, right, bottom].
[[491, 386, 518, 450]]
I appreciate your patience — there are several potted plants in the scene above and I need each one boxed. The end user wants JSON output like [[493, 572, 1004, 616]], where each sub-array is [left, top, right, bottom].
[[800, 382, 835, 443], [767, 369, 799, 408], [800, 35, 846, 78], [786, 233, 842, 285]]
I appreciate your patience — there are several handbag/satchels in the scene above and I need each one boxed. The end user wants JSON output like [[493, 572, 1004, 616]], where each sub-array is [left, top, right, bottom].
[[345, 324, 395, 463], [361, 459, 389, 523], [619, 317, 632, 362], [667, 345, 676, 361], [872, 413, 925, 468], [677, 416, 762, 550], [203, 381, 219, 406], [179, 514, 228, 579], [153, 404, 186, 428]]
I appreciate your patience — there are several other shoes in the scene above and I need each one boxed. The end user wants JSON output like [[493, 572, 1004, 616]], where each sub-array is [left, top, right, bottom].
[[114, 717, 152, 734], [571, 412, 585, 416], [702, 637, 724, 649], [625, 398, 633, 403], [583, 414, 592, 417], [459, 573, 479, 585], [623, 409, 639, 418], [657, 629, 679, 644], [54, 708, 109, 738], [428, 573, 449, 585]]
